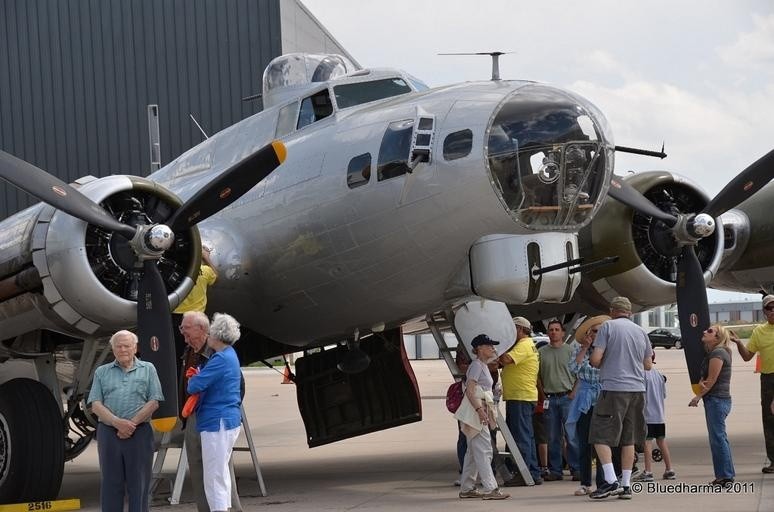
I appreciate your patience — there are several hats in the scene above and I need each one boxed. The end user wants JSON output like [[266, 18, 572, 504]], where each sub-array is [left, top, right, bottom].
[[610, 296, 632, 313], [762, 295, 774, 307], [471, 334, 499, 348], [512, 317, 531, 329], [576, 315, 613, 345]]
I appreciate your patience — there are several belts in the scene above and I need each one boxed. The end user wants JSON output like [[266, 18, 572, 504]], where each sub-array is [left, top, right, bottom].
[[547, 390, 570, 398]]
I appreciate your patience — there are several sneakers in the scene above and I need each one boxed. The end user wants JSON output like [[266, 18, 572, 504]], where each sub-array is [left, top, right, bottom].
[[663, 472, 676, 480], [619, 486, 633, 499], [633, 471, 655, 482], [575, 485, 592, 496], [483, 490, 512, 500], [459, 490, 482, 499], [590, 480, 625, 499], [544, 474, 563, 481], [762, 463, 774, 474], [707, 478, 735, 488], [619, 467, 639, 481]]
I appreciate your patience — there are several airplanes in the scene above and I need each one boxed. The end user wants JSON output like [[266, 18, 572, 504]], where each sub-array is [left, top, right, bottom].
[[0, 44, 774, 504]]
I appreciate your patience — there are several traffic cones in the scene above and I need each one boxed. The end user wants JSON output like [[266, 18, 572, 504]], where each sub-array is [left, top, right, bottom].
[[752, 350, 762, 375], [280, 363, 294, 386]]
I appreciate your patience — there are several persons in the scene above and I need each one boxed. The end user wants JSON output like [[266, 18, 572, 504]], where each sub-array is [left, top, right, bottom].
[[186, 313, 241, 512], [177, 310, 245, 512], [453, 297, 676, 500], [689, 324, 736, 488], [87, 329, 165, 512], [730, 295, 774, 473], [172, 246, 218, 365]]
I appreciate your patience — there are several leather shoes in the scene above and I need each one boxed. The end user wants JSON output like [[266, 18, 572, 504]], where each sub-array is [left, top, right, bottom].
[[505, 473, 541, 487]]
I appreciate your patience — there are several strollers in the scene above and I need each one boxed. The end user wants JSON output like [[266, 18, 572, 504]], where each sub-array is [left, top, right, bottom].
[[631, 440, 663, 464]]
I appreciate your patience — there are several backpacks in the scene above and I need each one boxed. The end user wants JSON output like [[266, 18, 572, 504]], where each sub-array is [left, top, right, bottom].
[[446, 381, 464, 414]]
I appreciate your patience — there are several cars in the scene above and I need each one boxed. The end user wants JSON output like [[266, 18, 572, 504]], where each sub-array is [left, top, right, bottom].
[[528, 331, 555, 349], [646, 325, 682, 350]]
